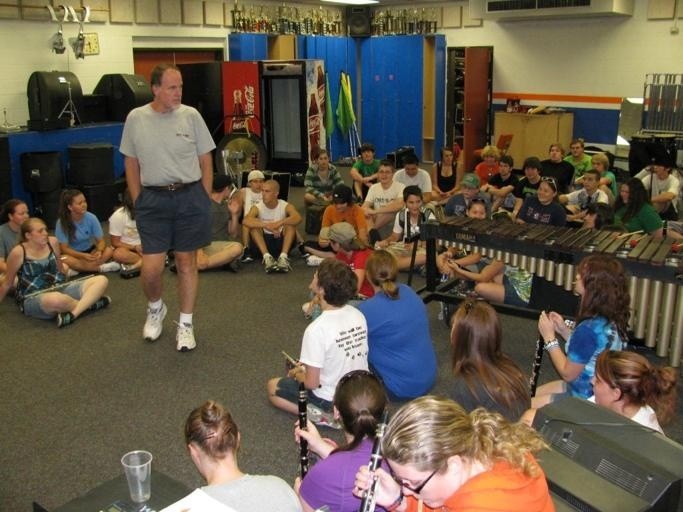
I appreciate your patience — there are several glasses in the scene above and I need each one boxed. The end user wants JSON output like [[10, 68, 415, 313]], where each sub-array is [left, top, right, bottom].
[[471, 197, 486, 203], [387, 467, 439, 494], [465, 295, 490, 312], [538, 174, 555, 183]]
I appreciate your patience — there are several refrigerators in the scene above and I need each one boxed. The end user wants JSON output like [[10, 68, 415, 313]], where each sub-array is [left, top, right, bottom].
[[259, 59, 327, 187]]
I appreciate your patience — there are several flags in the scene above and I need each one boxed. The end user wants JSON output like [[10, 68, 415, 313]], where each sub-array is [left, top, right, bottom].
[[322, 73, 335, 138], [335, 70, 355, 140]]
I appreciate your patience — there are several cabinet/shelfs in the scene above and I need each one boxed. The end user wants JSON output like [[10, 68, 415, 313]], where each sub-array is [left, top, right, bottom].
[[497, 114, 558, 165], [228, 32, 306, 61], [361, 35, 446, 164], [307, 36, 358, 163]]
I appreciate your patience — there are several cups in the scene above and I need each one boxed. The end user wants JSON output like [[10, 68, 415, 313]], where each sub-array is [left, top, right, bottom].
[[121, 450, 153, 503]]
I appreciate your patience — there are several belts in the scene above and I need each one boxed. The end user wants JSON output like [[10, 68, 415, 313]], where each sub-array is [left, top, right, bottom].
[[264, 232, 282, 239]]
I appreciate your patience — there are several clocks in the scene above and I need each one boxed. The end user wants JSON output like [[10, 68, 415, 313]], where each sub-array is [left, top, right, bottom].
[[81, 32, 100, 56]]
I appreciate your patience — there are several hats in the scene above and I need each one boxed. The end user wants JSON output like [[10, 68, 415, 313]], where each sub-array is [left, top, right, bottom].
[[522, 157, 540, 168], [460, 172, 479, 188], [358, 144, 375, 154], [320, 222, 358, 244], [248, 170, 264, 182], [480, 145, 500, 159], [331, 183, 351, 203], [247, 169, 264, 180], [591, 153, 608, 165], [314, 149, 328, 156], [402, 184, 421, 201], [142, 180, 200, 193]]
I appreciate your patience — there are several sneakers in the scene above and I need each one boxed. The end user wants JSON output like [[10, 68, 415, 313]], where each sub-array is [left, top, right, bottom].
[[88, 295, 112, 309], [306, 254, 323, 266], [243, 245, 252, 262], [57, 312, 74, 329], [173, 321, 197, 353], [261, 255, 290, 273], [142, 304, 168, 340], [227, 259, 240, 273], [306, 403, 344, 430], [98, 261, 119, 273]]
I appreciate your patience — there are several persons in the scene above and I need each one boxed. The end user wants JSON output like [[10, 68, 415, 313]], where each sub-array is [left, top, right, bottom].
[[446, 212, 531, 307], [353, 395, 556, 512], [444, 174, 494, 221], [56, 189, 120, 277], [391, 151, 431, 209], [267, 258, 368, 429], [541, 142, 574, 194], [158, 399, 303, 512], [445, 298, 531, 424], [358, 161, 406, 242], [293, 369, 401, 512], [109, 188, 146, 277], [298, 184, 369, 261], [354, 250, 436, 404], [515, 177, 567, 229], [241, 180, 302, 273], [169, 175, 243, 275], [574, 153, 618, 202], [512, 156, 544, 226], [350, 143, 381, 205], [640, 156, 681, 220], [613, 176, 666, 236], [585, 349, 677, 438], [118, 61, 216, 354], [528, 254, 636, 408], [430, 147, 463, 207], [0, 198, 32, 298], [582, 201, 613, 233], [563, 138, 594, 188], [1, 217, 113, 327], [557, 169, 609, 227], [374, 185, 429, 279], [475, 145, 501, 188], [301, 223, 375, 318], [484, 155, 515, 213], [434, 200, 488, 287], [241, 169, 308, 262], [303, 150, 343, 204]]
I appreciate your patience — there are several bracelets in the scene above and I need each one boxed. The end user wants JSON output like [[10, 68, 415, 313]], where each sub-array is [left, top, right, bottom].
[[542, 339, 561, 352]]
